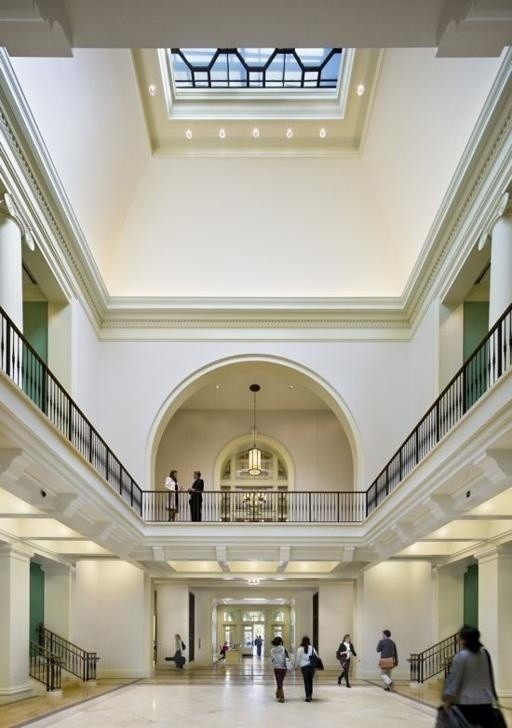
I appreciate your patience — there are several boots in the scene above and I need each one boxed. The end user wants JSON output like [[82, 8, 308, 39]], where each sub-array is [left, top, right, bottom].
[[276, 688, 285, 703]]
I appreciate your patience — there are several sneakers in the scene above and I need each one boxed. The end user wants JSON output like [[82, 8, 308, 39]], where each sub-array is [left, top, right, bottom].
[[305, 694, 313, 702]]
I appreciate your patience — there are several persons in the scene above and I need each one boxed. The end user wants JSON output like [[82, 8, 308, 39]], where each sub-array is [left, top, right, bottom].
[[441, 626, 497, 728], [256, 635, 262, 655], [188, 471, 204, 521], [271, 636, 289, 703], [294, 637, 319, 702], [175, 634, 183, 668], [336, 634, 356, 688], [218, 641, 228, 661], [164, 470, 180, 521], [376, 630, 397, 691]]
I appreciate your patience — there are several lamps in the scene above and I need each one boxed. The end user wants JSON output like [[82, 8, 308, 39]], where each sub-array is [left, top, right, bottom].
[[241, 381, 267, 478]]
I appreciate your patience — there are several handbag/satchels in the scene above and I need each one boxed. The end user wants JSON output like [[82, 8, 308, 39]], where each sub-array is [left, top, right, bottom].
[[336, 651, 347, 660], [492, 706, 508, 728], [380, 657, 396, 669], [309, 646, 324, 671], [285, 649, 293, 671]]
